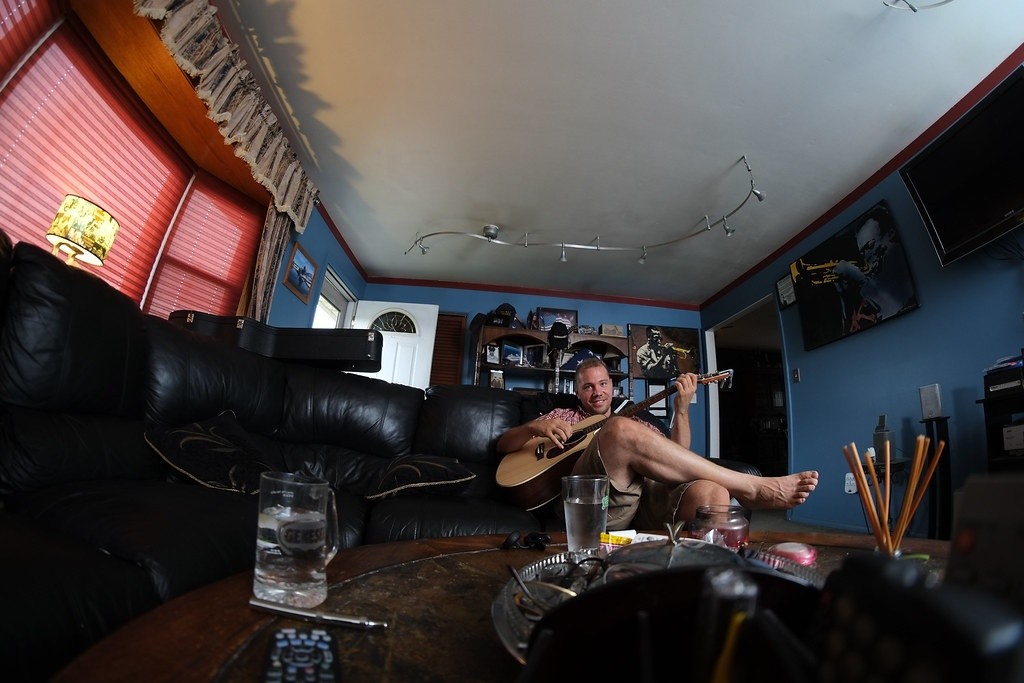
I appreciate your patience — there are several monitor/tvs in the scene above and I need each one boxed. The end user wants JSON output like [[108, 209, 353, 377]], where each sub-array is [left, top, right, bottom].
[[896, 63, 1024, 269]]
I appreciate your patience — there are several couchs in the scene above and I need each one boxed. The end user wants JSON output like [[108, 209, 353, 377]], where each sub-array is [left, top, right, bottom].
[[0, 229, 752, 683]]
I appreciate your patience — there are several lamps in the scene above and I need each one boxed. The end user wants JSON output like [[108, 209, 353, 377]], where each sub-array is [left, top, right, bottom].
[[44, 194, 121, 267], [638, 246, 647, 265], [722, 216, 736, 236], [603, 344, 624, 373], [559, 242, 567, 263], [419, 236, 430, 255], [752, 189, 767, 202]]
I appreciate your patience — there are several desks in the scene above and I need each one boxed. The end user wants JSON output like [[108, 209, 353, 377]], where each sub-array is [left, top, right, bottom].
[[857, 458, 914, 537], [57, 533, 950, 683]]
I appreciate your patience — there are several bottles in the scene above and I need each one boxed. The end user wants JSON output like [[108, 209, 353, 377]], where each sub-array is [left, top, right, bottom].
[[689, 504, 750, 548]]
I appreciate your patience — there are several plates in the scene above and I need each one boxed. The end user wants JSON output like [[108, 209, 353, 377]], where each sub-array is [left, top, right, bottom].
[[490, 546, 830, 660]]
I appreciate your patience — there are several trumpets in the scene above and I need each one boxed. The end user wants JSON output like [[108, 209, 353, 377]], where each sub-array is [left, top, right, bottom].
[[659, 344, 698, 361]]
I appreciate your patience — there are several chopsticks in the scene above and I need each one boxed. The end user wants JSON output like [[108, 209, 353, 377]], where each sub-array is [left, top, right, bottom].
[[840, 435, 945, 556]]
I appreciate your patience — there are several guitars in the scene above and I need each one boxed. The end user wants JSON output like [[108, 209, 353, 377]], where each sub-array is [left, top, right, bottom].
[[496, 367, 734, 513]]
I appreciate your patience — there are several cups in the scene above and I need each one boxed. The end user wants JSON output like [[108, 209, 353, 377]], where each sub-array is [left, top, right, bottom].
[[873, 431, 895, 462], [252, 470, 340, 609], [560, 474, 612, 552]]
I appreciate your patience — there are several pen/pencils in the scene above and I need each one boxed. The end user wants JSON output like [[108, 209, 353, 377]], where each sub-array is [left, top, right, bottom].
[[248, 597, 387, 631]]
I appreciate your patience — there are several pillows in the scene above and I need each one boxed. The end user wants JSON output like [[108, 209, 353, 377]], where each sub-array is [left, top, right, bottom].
[[365, 454, 477, 503], [143, 410, 280, 494]]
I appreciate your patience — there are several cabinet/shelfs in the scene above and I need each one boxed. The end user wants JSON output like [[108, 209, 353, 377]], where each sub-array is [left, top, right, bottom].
[[472, 324, 633, 402], [976, 366, 1023, 475]]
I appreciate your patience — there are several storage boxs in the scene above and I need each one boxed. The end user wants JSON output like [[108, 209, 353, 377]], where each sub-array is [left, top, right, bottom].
[[598, 324, 623, 335]]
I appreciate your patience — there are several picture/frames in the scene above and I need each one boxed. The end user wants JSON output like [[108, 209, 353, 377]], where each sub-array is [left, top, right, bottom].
[[627, 323, 700, 380], [790, 199, 922, 352], [483, 343, 501, 365], [514, 387, 543, 396], [501, 340, 523, 365], [524, 343, 546, 366], [488, 370, 505, 390], [283, 241, 319, 306], [536, 307, 578, 333]]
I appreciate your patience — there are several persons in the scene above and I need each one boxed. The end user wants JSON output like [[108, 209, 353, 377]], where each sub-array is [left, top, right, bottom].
[[299, 266, 306, 275], [497, 357, 819, 530], [831, 207, 915, 322], [635, 325, 680, 377]]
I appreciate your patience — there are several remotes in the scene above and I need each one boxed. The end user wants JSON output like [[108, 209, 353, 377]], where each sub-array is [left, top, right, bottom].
[[260, 628, 341, 683]]
